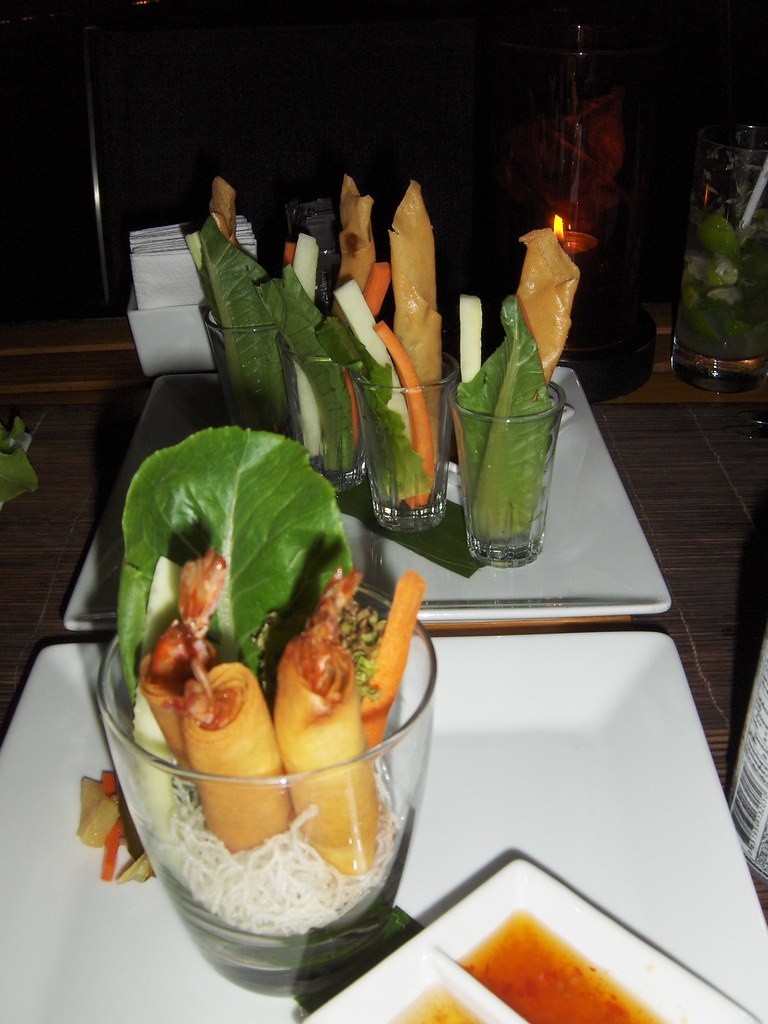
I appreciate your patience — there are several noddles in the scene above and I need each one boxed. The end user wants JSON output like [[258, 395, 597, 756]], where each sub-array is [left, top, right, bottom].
[[138, 787, 407, 936]]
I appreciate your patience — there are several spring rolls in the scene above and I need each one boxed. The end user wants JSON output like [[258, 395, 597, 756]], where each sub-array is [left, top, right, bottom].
[[136, 551, 234, 777], [178, 650, 291, 855], [277, 601, 394, 875], [91, 155, 587, 571], [320, 572, 427, 757]]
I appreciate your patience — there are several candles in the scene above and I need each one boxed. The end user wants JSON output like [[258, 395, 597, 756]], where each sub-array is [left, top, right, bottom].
[[549, 214, 600, 310]]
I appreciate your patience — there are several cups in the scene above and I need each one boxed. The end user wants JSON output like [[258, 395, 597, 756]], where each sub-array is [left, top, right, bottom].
[[96, 582, 437, 995], [276, 319, 368, 494], [350, 347, 463, 533], [672, 123, 768, 391], [200, 298, 296, 439], [127, 280, 217, 378], [448, 371, 566, 568]]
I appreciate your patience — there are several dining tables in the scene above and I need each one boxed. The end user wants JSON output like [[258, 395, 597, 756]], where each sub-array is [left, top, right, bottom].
[[5, 259, 768, 1023]]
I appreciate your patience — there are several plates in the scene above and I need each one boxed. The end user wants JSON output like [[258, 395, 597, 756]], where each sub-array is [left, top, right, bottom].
[[63, 365, 672, 621], [0, 632, 766, 1024], [296, 856, 761, 1024]]
